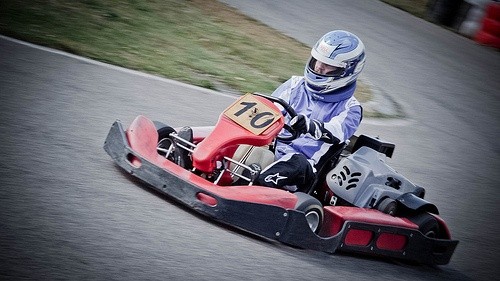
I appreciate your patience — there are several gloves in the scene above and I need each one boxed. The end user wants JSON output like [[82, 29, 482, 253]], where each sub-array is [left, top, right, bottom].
[[290, 113, 324, 140]]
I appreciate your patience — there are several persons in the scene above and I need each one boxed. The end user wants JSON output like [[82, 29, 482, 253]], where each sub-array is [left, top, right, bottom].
[[174, 28, 367, 193]]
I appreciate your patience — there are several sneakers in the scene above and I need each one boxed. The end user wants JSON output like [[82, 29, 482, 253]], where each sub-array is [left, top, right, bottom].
[[174, 126, 193, 171], [231, 163, 262, 186]]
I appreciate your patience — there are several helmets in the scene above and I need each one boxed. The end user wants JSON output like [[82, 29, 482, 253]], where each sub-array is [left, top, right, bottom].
[[304, 30, 366, 94]]
